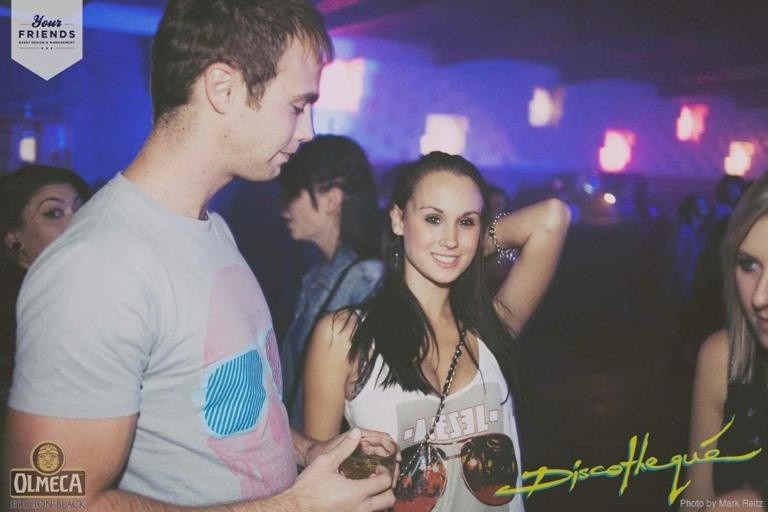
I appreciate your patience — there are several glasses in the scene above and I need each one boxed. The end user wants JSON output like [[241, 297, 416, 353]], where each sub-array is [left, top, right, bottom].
[[386, 431, 518, 510]]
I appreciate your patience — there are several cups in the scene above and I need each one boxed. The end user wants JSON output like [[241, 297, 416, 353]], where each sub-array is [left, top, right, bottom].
[[335, 436, 400, 484]]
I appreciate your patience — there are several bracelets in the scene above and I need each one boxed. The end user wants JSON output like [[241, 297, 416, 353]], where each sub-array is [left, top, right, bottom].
[[489, 210, 519, 262]]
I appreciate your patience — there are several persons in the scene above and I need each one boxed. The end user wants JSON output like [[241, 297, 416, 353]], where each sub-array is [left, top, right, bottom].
[[0, 1, 403, 510], [680, 170, 766, 511], [303, 152, 570, 510], [280, 134, 390, 437], [0, 165, 95, 420], [484, 169, 744, 238]]
